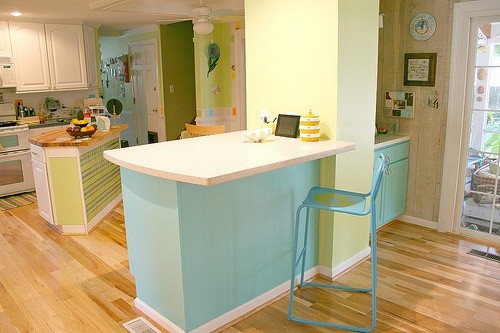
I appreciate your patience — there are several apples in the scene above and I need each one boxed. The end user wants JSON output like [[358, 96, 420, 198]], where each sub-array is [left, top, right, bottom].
[[67, 126, 80, 131]]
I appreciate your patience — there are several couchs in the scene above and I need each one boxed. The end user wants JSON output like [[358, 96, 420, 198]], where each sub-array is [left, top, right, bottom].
[[471, 160, 500, 203]]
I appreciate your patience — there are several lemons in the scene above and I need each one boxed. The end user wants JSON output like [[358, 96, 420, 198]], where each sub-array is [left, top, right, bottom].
[[72, 119, 88, 124], [80, 125, 95, 132]]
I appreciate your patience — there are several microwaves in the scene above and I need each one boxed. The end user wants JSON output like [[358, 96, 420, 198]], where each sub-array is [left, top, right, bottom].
[[0, 58, 16, 89]]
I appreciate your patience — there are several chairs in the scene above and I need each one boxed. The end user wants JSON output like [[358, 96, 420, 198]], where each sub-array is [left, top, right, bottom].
[[185, 123, 225, 138]]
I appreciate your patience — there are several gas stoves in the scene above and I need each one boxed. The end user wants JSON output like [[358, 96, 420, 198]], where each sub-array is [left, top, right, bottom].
[[0, 102, 28, 131]]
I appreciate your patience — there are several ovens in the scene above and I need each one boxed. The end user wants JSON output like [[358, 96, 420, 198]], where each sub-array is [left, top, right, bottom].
[[0, 125, 35, 199]]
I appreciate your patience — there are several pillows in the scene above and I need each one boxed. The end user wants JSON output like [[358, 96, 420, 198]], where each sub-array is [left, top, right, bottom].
[[489, 160, 500, 175]]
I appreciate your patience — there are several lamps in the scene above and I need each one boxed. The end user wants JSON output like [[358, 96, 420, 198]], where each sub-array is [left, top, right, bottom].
[[192, 17, 214, 34]]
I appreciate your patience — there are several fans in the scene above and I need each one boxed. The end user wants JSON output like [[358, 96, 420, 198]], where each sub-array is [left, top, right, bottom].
[[154, 0, 238, 22]]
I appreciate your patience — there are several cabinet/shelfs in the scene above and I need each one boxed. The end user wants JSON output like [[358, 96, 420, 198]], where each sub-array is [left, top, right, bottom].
[[374, 140, 410, 229], [0, 21, 98, 94], [27, 132, 129, 237]]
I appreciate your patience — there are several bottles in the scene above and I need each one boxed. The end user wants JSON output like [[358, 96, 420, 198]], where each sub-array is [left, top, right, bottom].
[[84, 112, 91, 126], [298, 110, 320, 142], [0, 93, 3, 103], [16, 101, 45, 123]]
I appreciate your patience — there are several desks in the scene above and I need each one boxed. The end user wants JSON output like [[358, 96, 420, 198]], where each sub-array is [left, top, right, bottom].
[[103, 130, 355, 333]]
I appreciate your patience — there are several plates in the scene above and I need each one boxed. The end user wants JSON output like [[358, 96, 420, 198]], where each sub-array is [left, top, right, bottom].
[[74, 123, 88, 128], [66, 129, 97, 139], [409, 13, 437, 40]]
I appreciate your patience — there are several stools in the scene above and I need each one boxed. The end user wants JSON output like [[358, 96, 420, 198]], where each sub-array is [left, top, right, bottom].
[[288, 153, 386, 332]]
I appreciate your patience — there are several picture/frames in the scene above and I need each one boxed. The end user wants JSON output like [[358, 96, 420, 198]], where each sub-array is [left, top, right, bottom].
[[275, 114, 301, 138], [409, 13, 436, 41]]
